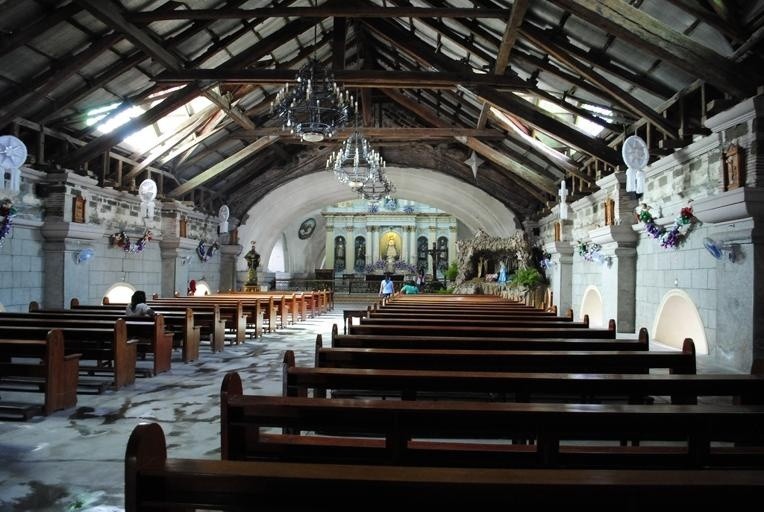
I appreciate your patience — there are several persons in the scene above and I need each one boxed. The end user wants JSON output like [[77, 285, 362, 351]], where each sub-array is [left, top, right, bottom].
[[400, 280, 419, 294], [496, 260, 507, 283], [379, 274, 395, 298], [244, 245, 260, 285], [335, 238, 448, 271], [126, 291, 156, 317]]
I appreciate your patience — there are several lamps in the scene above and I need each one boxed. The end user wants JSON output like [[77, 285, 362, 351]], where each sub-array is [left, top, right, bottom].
[[269, 0, 397, 204]]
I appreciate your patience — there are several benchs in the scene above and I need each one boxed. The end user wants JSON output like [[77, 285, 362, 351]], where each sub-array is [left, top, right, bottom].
[[0, 289, 136, 419], [122, 288, 761, 512]]
